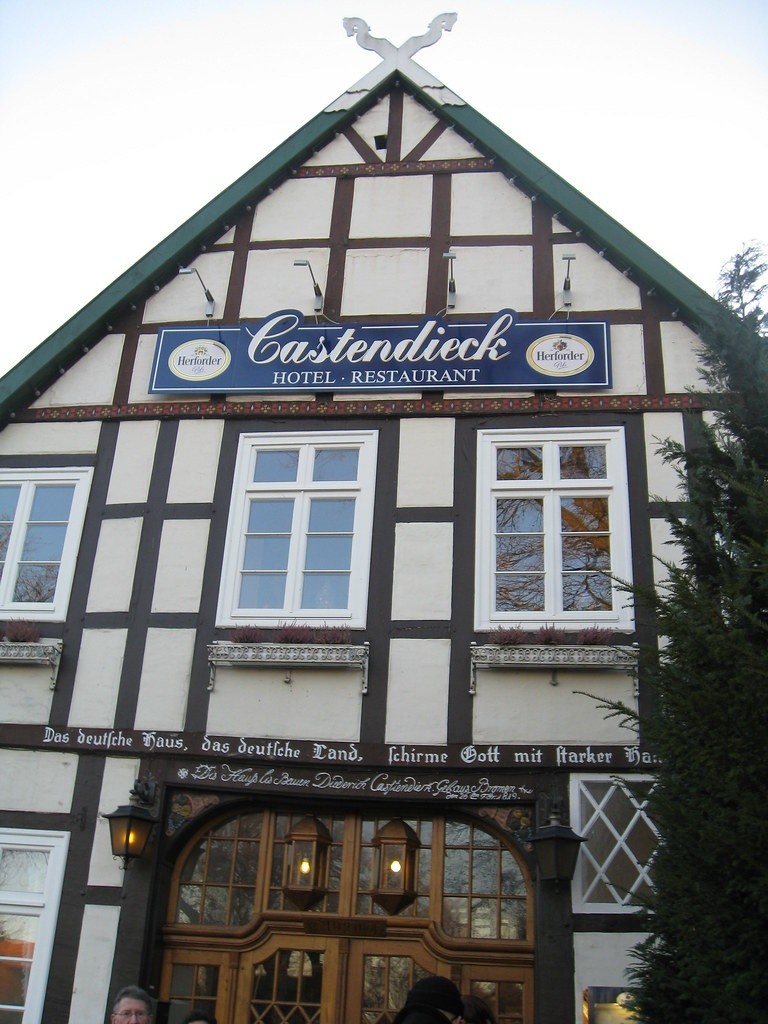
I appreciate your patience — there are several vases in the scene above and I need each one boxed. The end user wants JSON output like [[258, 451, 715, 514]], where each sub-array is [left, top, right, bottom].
[[0, 639, 63, 690], [469, 642, 640, 697], [206, 640, 371, 693]]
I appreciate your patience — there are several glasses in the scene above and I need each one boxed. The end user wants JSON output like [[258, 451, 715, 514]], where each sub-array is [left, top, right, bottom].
[[116, 1011, 146, 1018]]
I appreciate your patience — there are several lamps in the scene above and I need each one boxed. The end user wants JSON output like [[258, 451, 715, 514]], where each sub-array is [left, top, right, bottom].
[[442, 253, 456, 308], [370, 817, 421, 915], [526, 803, 588, 894], [281, 813, 332, 910], [179, 268, 215, 317], [294, 260, 323, 312], [562, 254, 576, 306], [102, 778, 161, 869]]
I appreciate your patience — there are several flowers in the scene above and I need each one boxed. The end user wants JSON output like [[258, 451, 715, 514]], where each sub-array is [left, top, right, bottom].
[[0, 617, 40, 642], [229, 620, 352, 645], [487, 623, 615, 646]]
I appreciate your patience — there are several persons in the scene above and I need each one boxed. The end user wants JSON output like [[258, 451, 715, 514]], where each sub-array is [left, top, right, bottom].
[[461, 994, 497, 1024], [184, 1009, 217, 1024], [393, 976, 466, 1024], [111, 985, 154, 1024]]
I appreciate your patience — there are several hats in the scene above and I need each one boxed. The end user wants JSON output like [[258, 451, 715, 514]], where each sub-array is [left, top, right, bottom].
[[406, 974, 464, 1017]]
